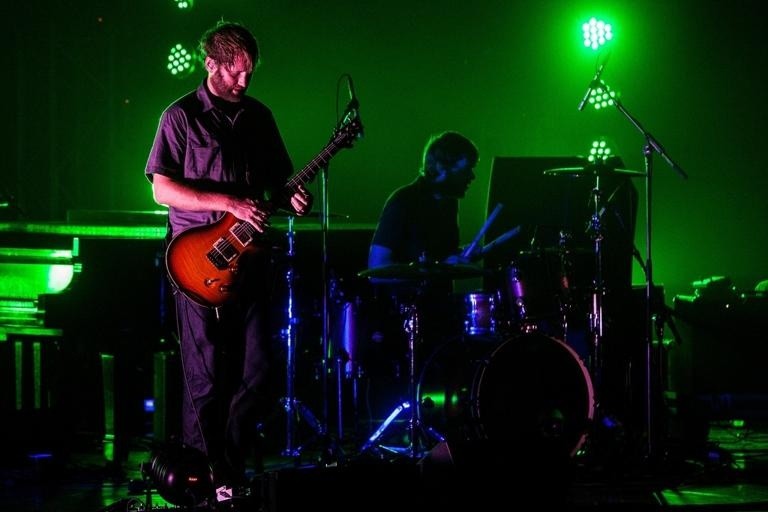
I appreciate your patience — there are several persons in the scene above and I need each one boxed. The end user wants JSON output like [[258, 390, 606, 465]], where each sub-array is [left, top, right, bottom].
[[366, 133, 478, 377], [141, 22, 314, 468]]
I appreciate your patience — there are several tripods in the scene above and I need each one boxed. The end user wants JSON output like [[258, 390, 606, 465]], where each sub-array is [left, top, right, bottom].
[[281, 216, 446, 463]]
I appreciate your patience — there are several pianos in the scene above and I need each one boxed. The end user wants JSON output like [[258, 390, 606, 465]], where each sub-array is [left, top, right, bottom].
[[0, 218, 380, 465]]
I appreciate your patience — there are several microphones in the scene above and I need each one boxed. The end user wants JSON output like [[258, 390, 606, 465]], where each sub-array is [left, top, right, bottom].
[[346, 79, 363, 138], [578, 49, 612, 112]]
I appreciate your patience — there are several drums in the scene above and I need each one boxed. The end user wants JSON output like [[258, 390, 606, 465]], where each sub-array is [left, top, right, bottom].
[[416, 323, 597, 456], [484, 251, 575, 332], [461, 289, 511, 330]]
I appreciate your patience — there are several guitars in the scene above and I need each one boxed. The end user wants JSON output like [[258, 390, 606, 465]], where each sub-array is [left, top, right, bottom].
[[165, 115, 360, 309]]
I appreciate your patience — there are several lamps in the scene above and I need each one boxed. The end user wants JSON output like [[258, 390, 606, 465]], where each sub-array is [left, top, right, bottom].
[[132, 443, 216, 510]]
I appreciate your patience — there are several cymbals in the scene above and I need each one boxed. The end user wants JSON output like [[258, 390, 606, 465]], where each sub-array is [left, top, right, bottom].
[[348, 261, 481, 282], [542, 167, 646, 178]]
